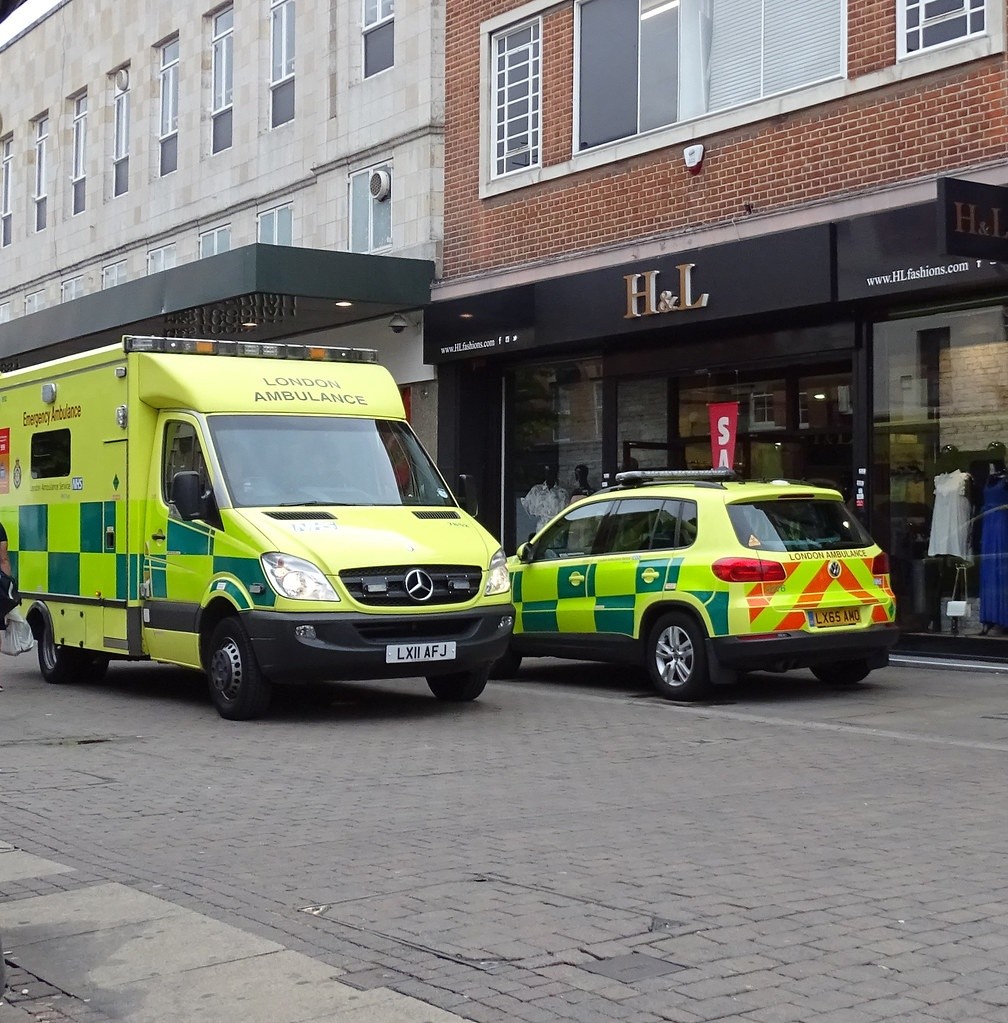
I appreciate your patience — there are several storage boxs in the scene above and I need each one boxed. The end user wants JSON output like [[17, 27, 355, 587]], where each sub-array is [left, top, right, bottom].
[[941, 596, 1002, 636]]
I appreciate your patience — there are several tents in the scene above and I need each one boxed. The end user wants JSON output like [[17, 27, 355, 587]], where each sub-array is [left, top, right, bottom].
[[0, 243, 435, 373]]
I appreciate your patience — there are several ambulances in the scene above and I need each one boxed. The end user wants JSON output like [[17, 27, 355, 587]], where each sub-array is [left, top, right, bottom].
[[505, 468, 896, 702], [0, 336, 514, 714]]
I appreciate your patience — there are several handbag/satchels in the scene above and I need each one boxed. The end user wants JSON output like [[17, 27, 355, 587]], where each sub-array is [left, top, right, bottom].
[[947, 600, 973, 618], [1, 608, 37, 656]]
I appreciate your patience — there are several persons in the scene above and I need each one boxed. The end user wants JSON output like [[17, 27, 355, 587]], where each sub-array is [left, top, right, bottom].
[[521, 459, 597, 548], [0, 522, 11, 692], [926, 441, 1008, 636]]
